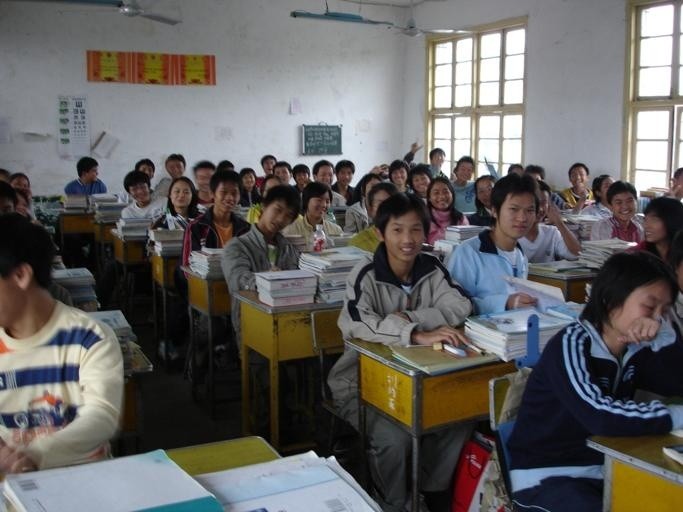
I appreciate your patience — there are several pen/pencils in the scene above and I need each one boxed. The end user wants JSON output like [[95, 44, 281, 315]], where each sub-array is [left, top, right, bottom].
[[468, 344, 486, 356], [422, 243, 433, 247]]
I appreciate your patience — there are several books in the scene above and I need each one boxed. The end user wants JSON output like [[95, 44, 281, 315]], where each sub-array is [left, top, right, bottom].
[[659, 443, 681, 466], [3, 448, 227, 512]]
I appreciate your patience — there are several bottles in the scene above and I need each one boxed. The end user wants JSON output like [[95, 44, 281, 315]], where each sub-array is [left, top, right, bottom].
[[324, 207, 336, 224], [314, 224, 325, 255]]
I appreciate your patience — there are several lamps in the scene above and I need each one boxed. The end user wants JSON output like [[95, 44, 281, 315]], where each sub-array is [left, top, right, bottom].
[[289, 1, 396, 27]]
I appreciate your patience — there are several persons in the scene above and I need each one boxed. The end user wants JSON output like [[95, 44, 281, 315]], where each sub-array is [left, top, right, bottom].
[[323, 191, 476, 512], [0, 145, 682, 427], [0, 209, 129, 478], [497, 248, 682, 512]]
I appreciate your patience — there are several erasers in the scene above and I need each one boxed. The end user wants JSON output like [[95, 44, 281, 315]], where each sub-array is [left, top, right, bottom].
[[432, 342, 443, 350]]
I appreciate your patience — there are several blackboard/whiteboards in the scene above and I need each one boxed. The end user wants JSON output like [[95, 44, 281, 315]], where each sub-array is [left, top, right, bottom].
[[302, 123, 343, 156]]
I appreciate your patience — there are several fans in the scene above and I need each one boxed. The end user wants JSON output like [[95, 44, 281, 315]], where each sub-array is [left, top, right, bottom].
[[366, 0, 475, 37], [55, 1, 185, 28]]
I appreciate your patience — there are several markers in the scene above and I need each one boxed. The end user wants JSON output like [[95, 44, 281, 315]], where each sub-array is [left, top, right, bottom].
[[444, 343, 467, 357]]
